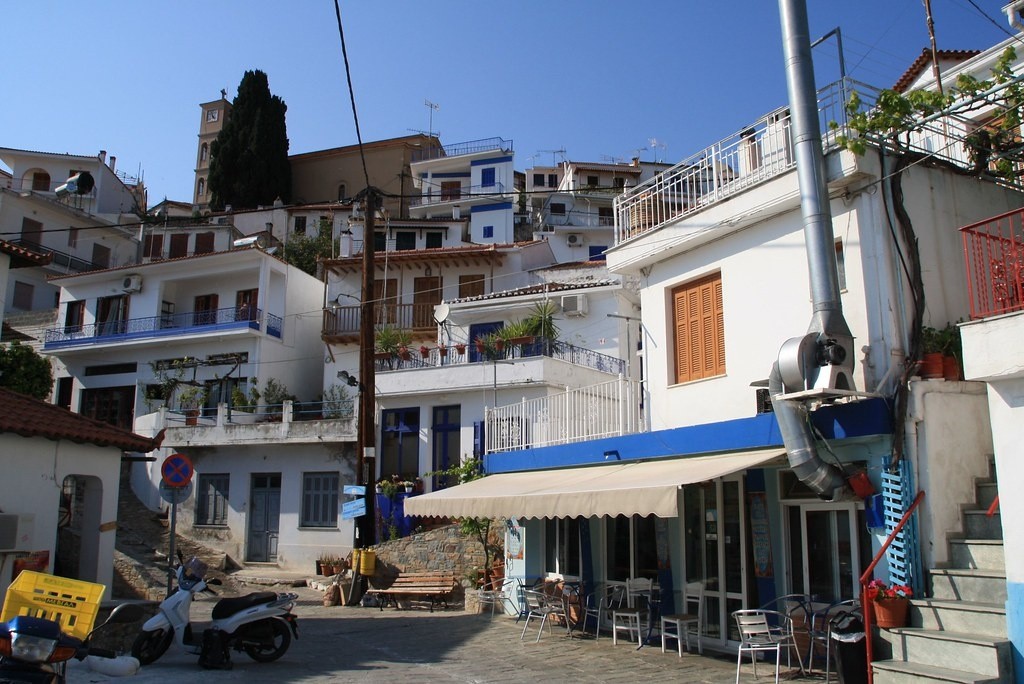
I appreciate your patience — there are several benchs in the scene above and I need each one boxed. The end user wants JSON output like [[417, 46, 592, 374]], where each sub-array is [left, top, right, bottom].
[[366, 571, 455, 613]]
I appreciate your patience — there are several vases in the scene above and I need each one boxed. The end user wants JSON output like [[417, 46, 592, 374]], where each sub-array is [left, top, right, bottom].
[[489, 559, 505, 592], [402, 354, 410, 360], [422, 352, 428, 358], [477, 345, 484, 352], [872, 599, 908, 628], [496, 343, 503, 350], [375, 483, 423, 493]]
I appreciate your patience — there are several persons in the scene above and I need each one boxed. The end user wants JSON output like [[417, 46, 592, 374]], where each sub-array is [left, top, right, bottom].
[[239, 302, 255, 321]]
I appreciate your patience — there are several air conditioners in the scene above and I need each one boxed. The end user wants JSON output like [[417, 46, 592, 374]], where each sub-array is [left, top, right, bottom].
[[121, 274, 142, 293], [561, 294, 588, 315], [567, 233, 584, 246]]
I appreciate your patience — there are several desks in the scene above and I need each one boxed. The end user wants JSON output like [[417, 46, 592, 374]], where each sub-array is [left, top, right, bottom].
[[636, 590, 681, 651], [562, 582, 606, 636]]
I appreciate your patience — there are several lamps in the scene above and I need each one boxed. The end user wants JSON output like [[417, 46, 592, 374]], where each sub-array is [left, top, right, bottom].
[[328, 291, 360, 311]]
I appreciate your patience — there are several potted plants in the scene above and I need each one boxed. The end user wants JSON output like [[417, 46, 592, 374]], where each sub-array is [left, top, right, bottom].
[[180, 387, 208, 425], [374, 327, 412, 358], [500, 319, 533, 344], [230, 376, 261, 424], [265, 378, 302, 422], [316, 552, 352, 576], [916, 323, 964, 381]]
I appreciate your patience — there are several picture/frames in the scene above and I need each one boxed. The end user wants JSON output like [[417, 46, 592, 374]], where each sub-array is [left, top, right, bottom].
[[458, 349, 465, 353], [440, 350, 447, 356]]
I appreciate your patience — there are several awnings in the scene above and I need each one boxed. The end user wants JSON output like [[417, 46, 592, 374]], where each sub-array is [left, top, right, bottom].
[[404, 447, 789, 521]]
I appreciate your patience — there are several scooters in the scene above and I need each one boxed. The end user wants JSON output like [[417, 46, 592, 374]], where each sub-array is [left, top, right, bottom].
[[130, 547, 300, 666], [0, 602, 143, 684]]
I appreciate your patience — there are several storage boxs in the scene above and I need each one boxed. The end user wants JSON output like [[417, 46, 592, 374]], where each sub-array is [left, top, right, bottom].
[[0, 569, 106, 641]]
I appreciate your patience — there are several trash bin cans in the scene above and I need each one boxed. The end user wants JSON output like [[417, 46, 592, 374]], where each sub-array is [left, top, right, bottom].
[[828, 610, 869, 684]]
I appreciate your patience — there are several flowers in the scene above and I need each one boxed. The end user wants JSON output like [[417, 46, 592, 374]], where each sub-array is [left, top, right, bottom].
[[438, 343, 447, 351], [866, 579, 912, 600], [495, 338, 502, 343], [375, 474, 421, 487], [474, 337, 483, 345], [420, 346, 429, 352], [455, 343, 465, 349], [397, 347, 410, 357]]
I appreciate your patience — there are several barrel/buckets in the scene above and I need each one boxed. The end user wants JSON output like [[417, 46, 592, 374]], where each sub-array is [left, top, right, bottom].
[[352, 549, 360, 571], [360, 550, 375, 575]]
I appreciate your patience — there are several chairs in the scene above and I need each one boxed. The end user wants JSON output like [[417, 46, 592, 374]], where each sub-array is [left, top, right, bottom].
[[660, 581, 704, 657], [477, 577, 577, 642], [731, 594, 863, 684], [612, 579, 653, 646], [582, 584, 630, 640]]
